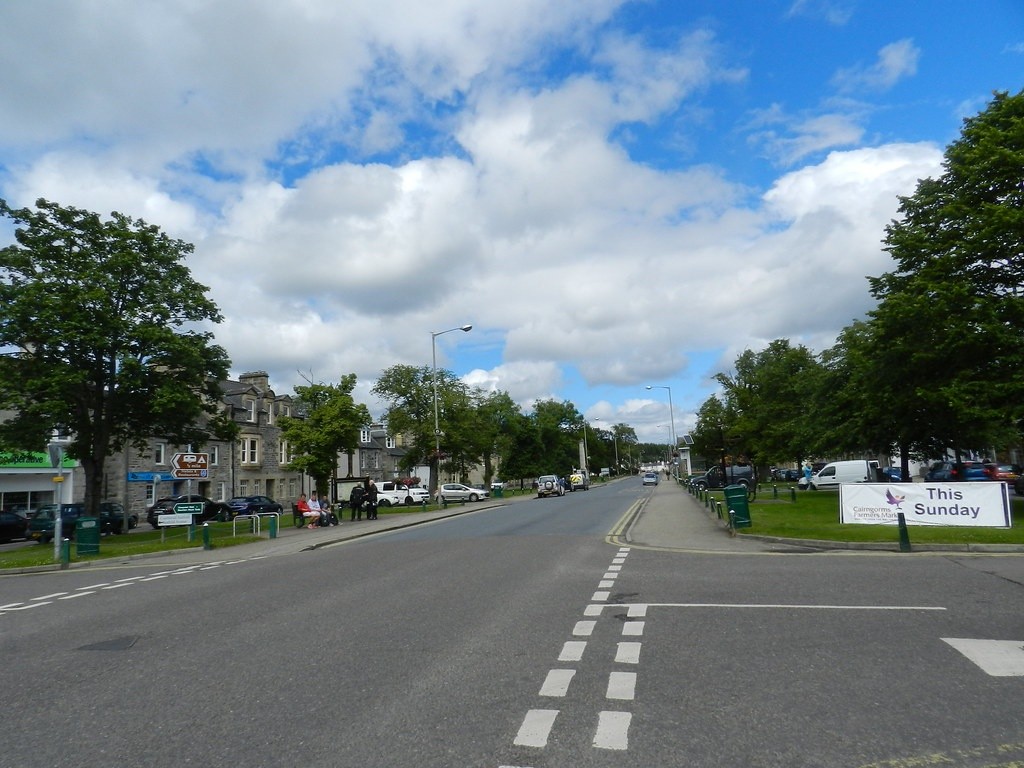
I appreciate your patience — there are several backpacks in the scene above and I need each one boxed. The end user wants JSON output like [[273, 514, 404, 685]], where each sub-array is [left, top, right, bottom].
[[330, 513, 338, 526], [318, 513, 329, 527]]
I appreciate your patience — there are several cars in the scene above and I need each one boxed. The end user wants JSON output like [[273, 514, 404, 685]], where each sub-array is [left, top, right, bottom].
[[26, 502, 139, 545], [226, 496, 284, 517], [362, 493, 400, 509], [769, 466, 798, 482], [0, 511, 30, 544], [642, 469, 659, 485], [433, 482, 489, 503], [879, 461, 1024, 495]]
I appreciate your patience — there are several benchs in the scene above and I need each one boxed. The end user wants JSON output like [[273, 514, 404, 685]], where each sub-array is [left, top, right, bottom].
[[290, 500, 333, 528]]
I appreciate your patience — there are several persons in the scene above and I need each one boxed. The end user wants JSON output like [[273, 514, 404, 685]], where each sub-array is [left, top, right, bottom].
[[350, 480, 378, 521], [982, 455, 991, 464], [298, 492, 340, 530], [736, 455, 752, 468], [784, 460, 819, 491], [557, 477, 568, 494], [661, 469, 670, 480]]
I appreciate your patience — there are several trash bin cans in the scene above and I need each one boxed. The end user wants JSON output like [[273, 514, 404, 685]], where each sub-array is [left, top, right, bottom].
[[723, 484, 752, 528], [76, 516, 100, 555], [494, 485, 501, 496]]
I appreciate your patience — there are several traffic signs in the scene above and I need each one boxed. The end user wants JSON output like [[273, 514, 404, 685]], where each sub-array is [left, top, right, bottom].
[[157, 503, 203, 526], [171, 453, 207, 479]]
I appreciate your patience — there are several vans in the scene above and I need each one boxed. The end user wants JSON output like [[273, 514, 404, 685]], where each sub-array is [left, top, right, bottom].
[[570, 474, 589, 491], [374, 481, 430, 505], [798, 460, 879, 490]]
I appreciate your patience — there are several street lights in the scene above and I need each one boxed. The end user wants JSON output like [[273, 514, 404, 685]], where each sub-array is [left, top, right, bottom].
[[432, 325, 471, 503], [584, 418, 600, 477], [645, 387, 679, 485]]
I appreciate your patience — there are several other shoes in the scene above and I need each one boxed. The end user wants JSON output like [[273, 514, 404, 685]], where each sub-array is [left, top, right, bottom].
[[339, 522, 343, 526], [367, 516, 377, 520], [307, 523, 317, 529], [357, 518, 362, 521], [329, 523, 334, 526], [350, 519, 355, 521]]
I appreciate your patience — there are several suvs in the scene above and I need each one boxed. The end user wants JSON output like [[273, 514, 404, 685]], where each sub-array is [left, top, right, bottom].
[[537, 476, 564, 497], [690, 466, 760, 489], [490, 480, 504, 491], [147, 493, 233, 530]]
